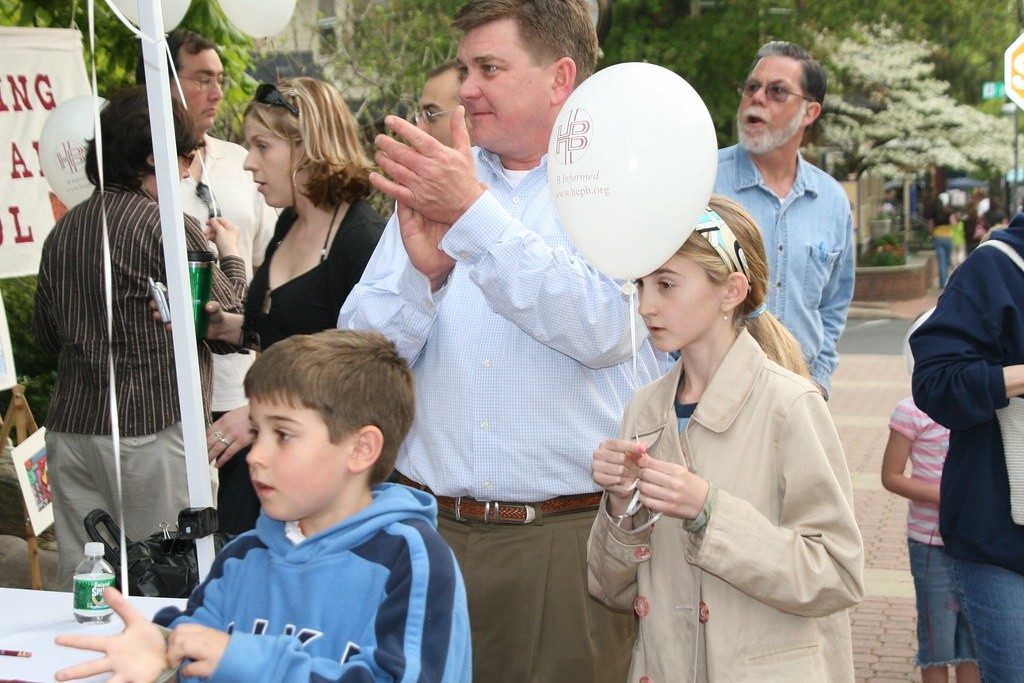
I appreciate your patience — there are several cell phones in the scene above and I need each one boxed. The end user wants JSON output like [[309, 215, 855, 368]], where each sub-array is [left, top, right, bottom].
[[147, 276, 171, 323]]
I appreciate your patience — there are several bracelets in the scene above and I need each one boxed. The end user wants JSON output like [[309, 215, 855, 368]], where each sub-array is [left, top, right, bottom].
[[682, 480, 718, 532]]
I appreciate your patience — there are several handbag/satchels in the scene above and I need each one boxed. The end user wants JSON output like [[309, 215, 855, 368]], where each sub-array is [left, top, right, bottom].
[[85, 501, 235, 598], [990, 396, 1024, 530]]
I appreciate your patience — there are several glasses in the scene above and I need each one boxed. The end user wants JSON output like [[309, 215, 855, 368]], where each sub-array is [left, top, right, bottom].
[[735, 81, 813, 110], [180, 152, 195, 167], [414, 106, 455, 123], [173, 74, 231, 94], [195, 181, 222, 219], [252, 82, 300, 120]]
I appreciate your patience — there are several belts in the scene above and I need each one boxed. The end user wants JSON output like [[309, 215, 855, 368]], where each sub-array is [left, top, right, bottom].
[[397, 474, 601, 525]]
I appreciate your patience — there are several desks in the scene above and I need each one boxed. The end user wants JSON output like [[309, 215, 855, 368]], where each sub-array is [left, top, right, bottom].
[[0, 588, 189, 683]]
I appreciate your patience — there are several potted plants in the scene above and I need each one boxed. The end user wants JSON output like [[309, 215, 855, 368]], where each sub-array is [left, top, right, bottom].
[[869, 211, 892, 238]]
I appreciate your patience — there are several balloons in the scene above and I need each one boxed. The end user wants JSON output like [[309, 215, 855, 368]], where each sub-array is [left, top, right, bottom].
[[218, 0, 297, 39], [39, 94, 112, 212], [550, 59, 721, 278], [112, 0, 190, 34]]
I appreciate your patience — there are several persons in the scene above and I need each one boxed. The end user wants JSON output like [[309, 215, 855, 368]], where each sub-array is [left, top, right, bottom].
[[16, 0, 672, 683], [585, 189, 864, 683], [710, 40, 856, 408], [877, 187, 1024, 682]]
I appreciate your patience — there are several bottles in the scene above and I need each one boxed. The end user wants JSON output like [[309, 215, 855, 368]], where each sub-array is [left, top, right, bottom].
[[72, 542, 115, 627]]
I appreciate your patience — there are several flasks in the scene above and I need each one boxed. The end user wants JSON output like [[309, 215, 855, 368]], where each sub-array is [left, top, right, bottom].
[[187, 251, 213, 341]]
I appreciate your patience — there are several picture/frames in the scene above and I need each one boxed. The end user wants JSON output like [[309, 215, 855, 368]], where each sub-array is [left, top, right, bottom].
[[11, 425, 55, 538]]
